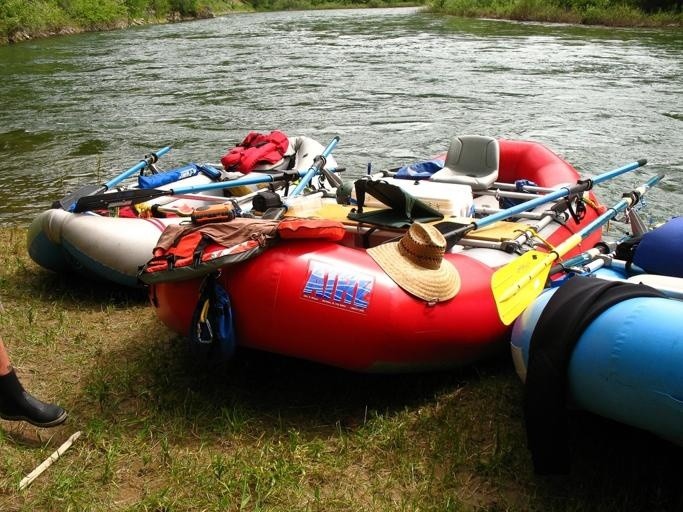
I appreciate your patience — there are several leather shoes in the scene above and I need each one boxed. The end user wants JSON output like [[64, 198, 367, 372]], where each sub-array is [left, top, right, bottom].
[[1, 368, 67, 427]]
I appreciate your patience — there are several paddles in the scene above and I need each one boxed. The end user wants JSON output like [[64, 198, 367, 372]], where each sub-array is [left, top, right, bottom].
[[261, 136, 340, 221], [59, 139, 173, 212], [74, 166, 346, 213], [433, 158, 648, 252], [490, 170, 666, 327]]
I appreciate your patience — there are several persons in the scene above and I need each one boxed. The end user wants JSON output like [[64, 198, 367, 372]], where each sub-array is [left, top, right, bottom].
[[0, 337, 68, 428]]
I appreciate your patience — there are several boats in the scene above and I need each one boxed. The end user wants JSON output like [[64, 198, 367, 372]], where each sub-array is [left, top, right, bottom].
[[511, 215, 683, 442], [150, 139, 602, 375], [27, 136, 344, 290]]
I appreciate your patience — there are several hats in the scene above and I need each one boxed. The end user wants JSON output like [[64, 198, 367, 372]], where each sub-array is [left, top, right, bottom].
[[366, 222, 461, 303]]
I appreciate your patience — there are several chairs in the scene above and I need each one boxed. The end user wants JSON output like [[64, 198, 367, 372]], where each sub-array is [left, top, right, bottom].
[[428, 134, 500, 190]]
